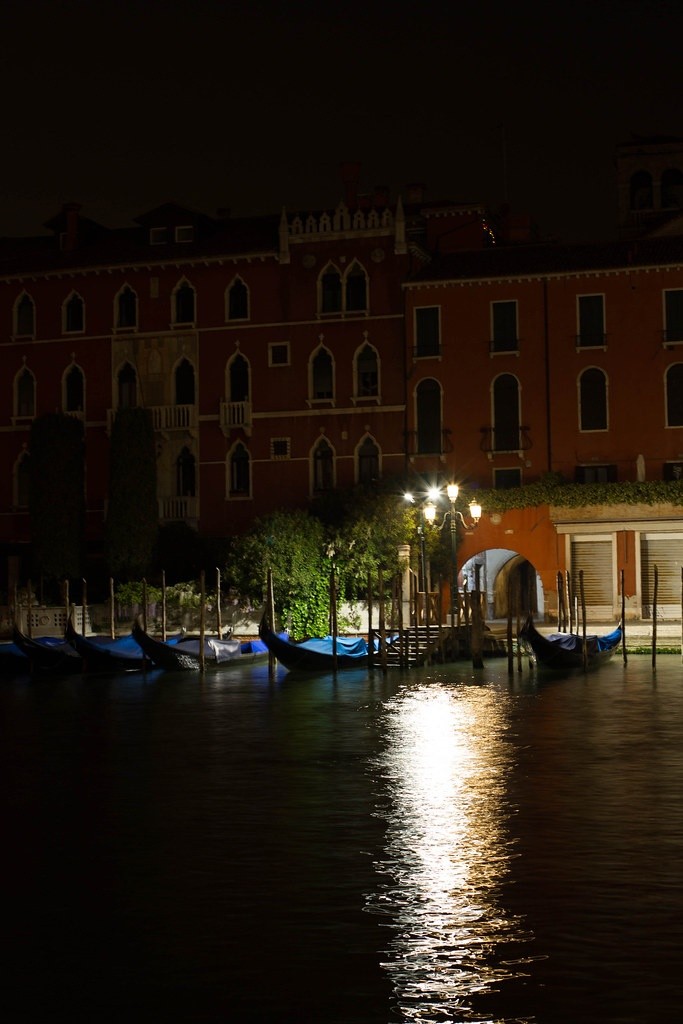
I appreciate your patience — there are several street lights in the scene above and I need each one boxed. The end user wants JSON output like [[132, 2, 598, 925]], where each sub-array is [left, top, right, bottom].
[[424, 480, 482, 611], [404, 491, 438, 609]]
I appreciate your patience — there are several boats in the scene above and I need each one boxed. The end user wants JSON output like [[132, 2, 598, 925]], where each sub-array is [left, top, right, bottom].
[[0, 637, 68, 661], [518, 612, 623, 669], [64, 608, 185, 668], [133, 615, 292, 670], [259, 611, 395, 669], [13, 623, 123, 664]]
